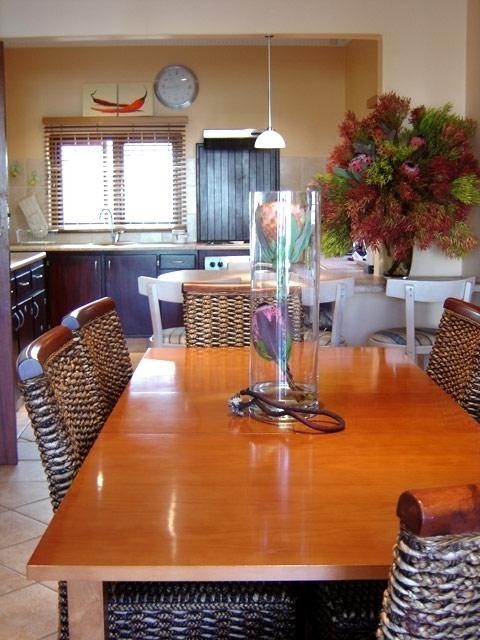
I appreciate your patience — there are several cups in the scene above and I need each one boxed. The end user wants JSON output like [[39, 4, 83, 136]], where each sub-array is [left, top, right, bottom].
[[367, 264, 374, 274]]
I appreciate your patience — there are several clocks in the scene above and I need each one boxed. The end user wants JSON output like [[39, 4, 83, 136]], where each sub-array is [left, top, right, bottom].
[[154, 64, 199, 110]]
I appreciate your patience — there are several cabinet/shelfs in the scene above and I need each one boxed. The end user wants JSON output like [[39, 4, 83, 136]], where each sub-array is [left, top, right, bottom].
[[157, 250, 198, 329], [45, 251, 158, 338], [11, 259, 46, 401]]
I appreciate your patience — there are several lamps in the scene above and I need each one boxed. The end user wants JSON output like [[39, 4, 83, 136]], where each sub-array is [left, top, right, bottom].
[[254, 35, 286, 149]]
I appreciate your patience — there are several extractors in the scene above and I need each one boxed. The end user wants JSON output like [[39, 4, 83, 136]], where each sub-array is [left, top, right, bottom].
[[205, 128, 275, 142]]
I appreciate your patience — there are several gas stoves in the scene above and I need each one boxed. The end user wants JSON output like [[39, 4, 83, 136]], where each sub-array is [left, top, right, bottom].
[[201, 241, 256, 269]]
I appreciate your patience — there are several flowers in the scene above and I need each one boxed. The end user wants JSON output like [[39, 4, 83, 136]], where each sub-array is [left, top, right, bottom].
[[306, 89, 479, 262], [248, 201, 316, 399]]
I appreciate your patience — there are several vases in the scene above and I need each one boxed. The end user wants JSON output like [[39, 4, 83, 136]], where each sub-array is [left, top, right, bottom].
[[372, 247, 413, 278]]
